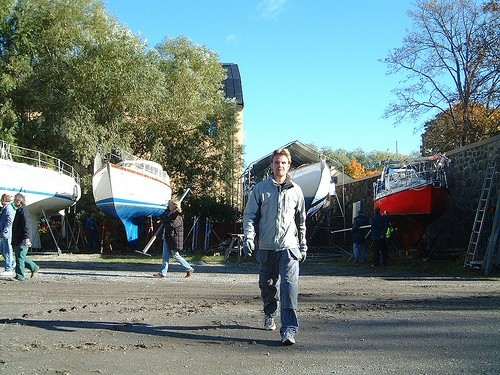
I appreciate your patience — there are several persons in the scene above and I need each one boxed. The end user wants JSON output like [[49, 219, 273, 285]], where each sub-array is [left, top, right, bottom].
[[350, 211, 369, 266], [370, 207, 387, 268], [243, 149, 306, 345], [85, 212, 101, 253], [9, 193, 39, 281], [0, 193, 15, 276], [153, 199, 194, 278]]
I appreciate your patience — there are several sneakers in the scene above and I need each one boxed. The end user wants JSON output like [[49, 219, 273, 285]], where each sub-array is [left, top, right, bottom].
[[281, 332, 296, 344], [263, 314, 277, 330]]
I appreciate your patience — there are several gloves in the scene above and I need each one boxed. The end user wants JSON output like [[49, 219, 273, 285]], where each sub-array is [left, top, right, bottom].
[[300, 251, 307, 263], [242, 239, 255, 257]]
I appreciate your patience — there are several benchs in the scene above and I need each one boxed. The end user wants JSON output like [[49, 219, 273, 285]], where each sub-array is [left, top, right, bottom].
[[223, 234, 245, 264]]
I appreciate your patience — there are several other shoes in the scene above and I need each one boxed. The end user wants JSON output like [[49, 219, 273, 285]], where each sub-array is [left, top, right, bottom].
[[31, 266, 39, 278], [185, 268, 194, 278], [6, 277, 19, 281], [153, 273, 163, 277]]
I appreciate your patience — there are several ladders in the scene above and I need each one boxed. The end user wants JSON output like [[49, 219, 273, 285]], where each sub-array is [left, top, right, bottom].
[[463, 163, 495, 268]]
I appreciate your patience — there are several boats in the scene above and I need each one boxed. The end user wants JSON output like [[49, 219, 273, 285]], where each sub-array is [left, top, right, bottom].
[[265, 160, 332, 221], [91, 146, 172, 249], [372, 154, 453, 250], [0, 139, 82, 250]]
